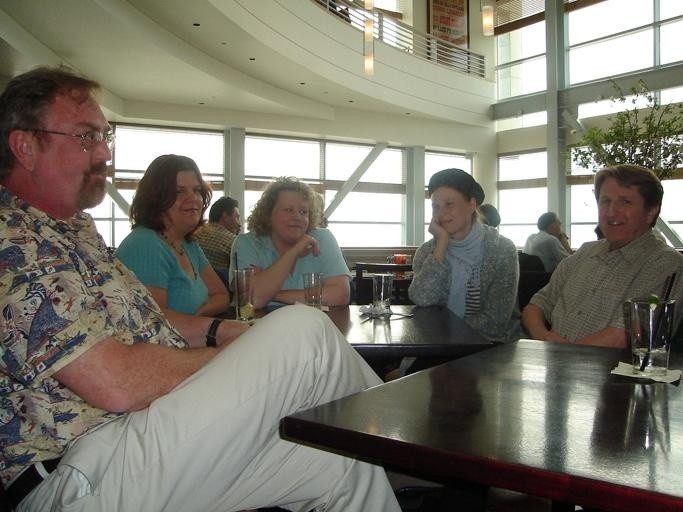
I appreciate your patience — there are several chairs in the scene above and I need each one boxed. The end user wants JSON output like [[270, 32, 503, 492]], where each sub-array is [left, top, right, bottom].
[[520, 271, 550, 313], [355, 262, 414, 306], [518, 254, 544, 272]]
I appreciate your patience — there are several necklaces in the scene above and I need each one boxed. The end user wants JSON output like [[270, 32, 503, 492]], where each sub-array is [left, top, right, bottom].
[[161, 232, 184, 256]]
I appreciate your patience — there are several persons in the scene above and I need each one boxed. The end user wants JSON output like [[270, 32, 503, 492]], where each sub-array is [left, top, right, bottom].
[[193, 194, 242, 274], [0, 65, 401, 512], [109, 153, 229, 318], [398, 168, 528, 378], [522, 212, 573, 272], [229, 176, 354, 313], [479, 202, 502, 228], [521, 164, 682, 352]]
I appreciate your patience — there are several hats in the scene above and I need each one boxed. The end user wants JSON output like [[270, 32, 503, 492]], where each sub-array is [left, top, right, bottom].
[[429, 169, 484, 206]]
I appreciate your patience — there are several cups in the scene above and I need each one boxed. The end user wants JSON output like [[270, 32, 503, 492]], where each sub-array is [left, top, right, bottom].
[[232, 269, 255, 321], [386, 254, 407, 278], [630, 299, 676, 376], [373, 314, 391, 344], [372, 274, 394, 314], [623, 383, 671, 456], [302, 272, 324, 310]]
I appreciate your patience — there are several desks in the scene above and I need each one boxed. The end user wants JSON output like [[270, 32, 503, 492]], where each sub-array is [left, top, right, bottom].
[[352, 273, 416, 289], [212, 303, 491, 377], [277, 338, 683, 511]]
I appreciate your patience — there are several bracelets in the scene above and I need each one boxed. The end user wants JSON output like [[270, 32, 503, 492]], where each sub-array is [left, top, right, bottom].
[[206, 319, 223, 347]]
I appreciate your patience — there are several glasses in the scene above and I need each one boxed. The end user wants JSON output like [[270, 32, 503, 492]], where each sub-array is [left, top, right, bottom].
[[12, 128, 115, 152]]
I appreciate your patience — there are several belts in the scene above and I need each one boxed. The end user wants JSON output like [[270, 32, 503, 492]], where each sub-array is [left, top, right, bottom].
[[6, 456, 62, 510]]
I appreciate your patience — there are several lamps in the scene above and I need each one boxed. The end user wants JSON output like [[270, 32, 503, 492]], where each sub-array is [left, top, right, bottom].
[[481, 4, 493, 37], [364, 0, 377, 73]]
